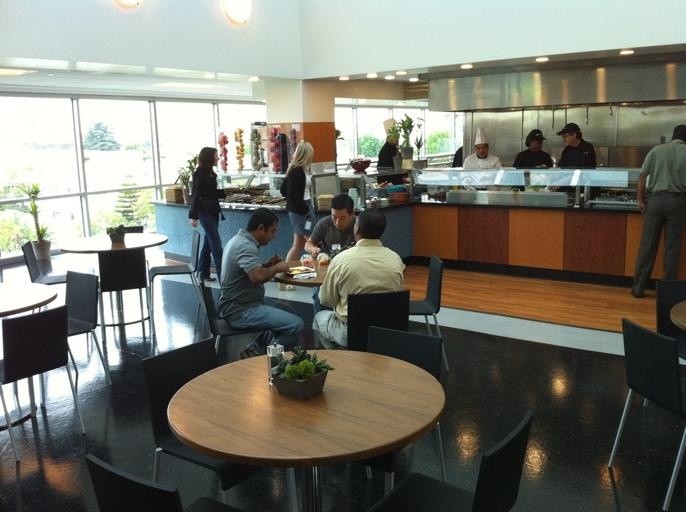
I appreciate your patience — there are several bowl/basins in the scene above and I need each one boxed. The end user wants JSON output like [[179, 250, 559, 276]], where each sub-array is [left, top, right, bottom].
[[351, 159, 371, 172]]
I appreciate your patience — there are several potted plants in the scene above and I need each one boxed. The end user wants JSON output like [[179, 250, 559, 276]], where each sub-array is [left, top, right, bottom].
[[19, 185, 51, 259], [107, 225, 124, 241], [175, 167, 190, 206], [188, 155, 199, 200], [274, 350, 328, 400], [388, 114, 423, 159]]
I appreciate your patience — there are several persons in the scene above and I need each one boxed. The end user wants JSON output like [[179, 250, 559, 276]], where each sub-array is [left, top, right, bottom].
[[375, 118, 410, 184], [512, 129, 554, 192], [279, 141, 316, 263], [550, 123, 603, 201], [630, 123, 686, 299], [215, 207, 305, 359], [188, 147, 226, 283], [304, 194, 361, 314], [311, 207, 406, 349], [463, 127, 505, 191]]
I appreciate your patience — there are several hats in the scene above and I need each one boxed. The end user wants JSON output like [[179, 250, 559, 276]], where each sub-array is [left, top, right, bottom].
[[474, 127, 489, 144], [557, 122, 581, 136], [524, 129, 548, 142], [383, 118, 400, 137]]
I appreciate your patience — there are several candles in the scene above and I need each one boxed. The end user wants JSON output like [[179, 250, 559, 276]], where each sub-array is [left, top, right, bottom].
[[271, 253, 328, 318]]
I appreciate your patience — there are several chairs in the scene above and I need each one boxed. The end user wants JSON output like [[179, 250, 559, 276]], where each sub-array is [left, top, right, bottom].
[[94, 248, 149, 347], [348, 292, 408, 356], [149, 230, 203, 314], [198, 274, 270, 352], [367, 408, 536, 511], [21, 242, 67, 314], [314, 327, 444, 495], [1, 306, 86, 462], [87, 452, 240, 511], [408, 256, 451, 373], [64, 270, 113, 384], [604, 319, 685, 512], [141, 337, 265, 502]]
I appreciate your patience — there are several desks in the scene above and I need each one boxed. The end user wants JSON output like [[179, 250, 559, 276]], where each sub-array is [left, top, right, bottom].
[[59, 233, 168, 348], [2, 280, 57, 409], [165, 349, 446, 511]]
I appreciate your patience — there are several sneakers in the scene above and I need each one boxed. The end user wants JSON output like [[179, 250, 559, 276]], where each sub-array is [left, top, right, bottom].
[[239, 348, 258, 360]]
